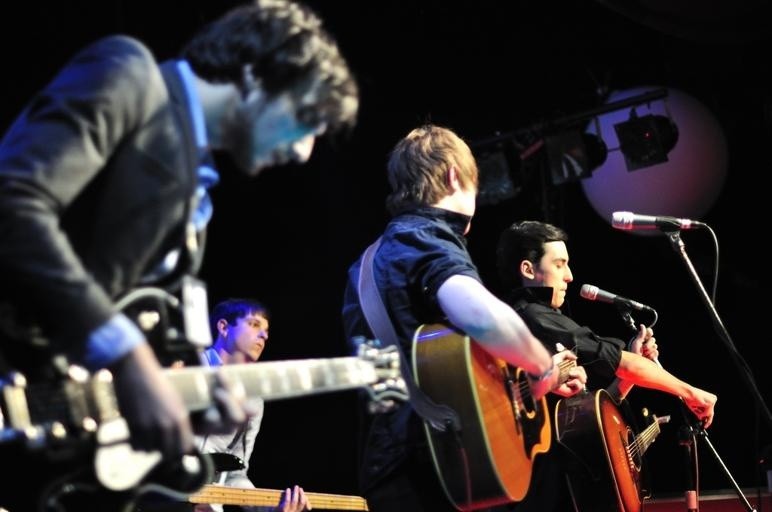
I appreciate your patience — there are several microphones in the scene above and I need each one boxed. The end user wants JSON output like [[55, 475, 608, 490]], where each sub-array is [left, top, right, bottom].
[[580, 284, 647, 312], [611, 211, 706, 230]]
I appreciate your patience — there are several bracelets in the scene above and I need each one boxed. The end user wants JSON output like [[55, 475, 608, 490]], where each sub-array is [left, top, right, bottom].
[[526, 356, 557, 383]]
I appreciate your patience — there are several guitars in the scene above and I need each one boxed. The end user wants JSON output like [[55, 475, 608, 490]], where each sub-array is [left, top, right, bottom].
[[553, 386, 671, 512], [63, 286, 410, 509], [138, 453, 369, 512], [412, 321, 579, 510]]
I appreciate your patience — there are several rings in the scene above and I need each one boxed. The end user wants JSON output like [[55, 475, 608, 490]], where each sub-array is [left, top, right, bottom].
[[156, 419, 178, 441]]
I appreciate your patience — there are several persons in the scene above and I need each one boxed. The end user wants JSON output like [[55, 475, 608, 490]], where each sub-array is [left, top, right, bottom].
[[336, 120, 592, 512], [486, 216, 721, 511], [175, 298, 319, 512], [1, 0, 365, 512]]
